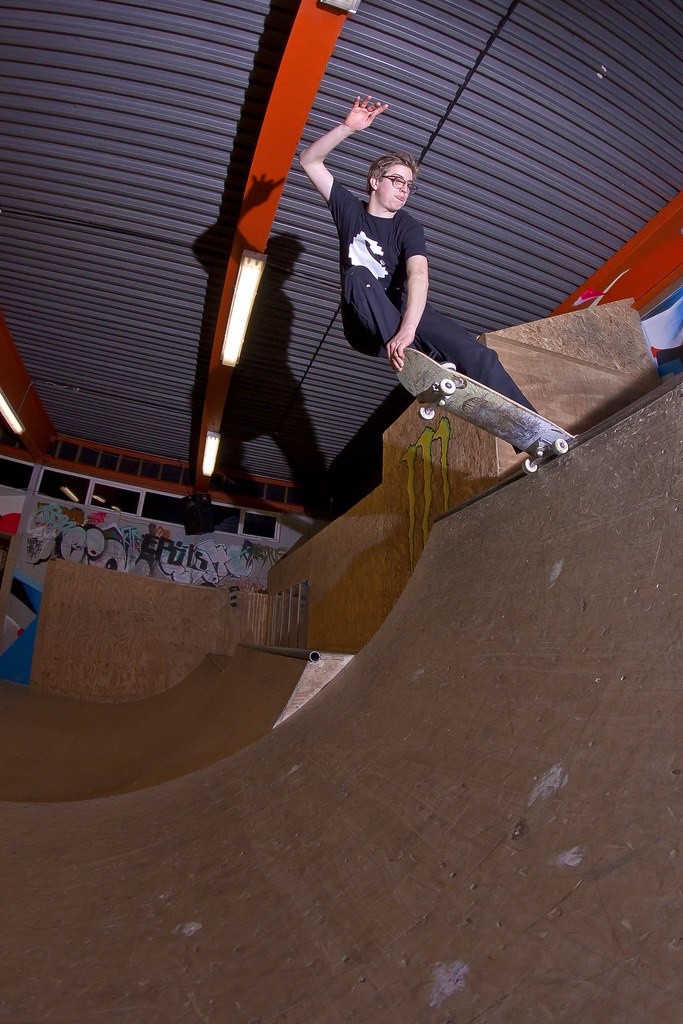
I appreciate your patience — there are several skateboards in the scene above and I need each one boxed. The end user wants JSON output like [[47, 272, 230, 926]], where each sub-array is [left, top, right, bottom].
[[394, 346, 575, 474]]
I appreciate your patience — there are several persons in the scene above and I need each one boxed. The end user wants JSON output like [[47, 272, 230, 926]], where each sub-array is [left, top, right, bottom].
[[299, 95, 541, 454]]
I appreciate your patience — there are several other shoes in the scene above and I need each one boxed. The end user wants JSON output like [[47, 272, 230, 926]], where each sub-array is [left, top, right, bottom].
[[439, 360, 457, 372]]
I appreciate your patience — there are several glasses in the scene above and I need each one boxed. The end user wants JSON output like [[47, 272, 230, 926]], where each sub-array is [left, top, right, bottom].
[[380, 175, 419, 195]]
